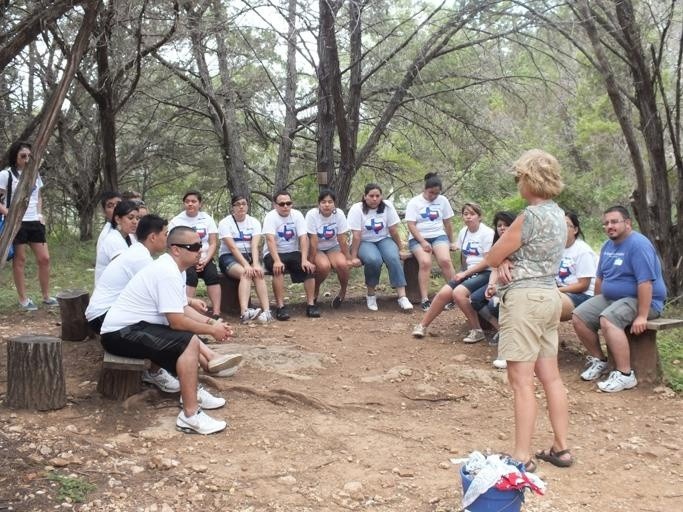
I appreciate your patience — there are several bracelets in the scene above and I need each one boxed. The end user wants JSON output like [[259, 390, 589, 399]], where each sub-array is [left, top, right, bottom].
[[206, 316, 217, 328], [486, 283, 494, 286], [186, 296, 193, 307]]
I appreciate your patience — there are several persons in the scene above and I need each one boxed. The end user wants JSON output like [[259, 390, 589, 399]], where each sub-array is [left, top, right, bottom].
[[482, 149, 574, 471], [412, 204, 495, 344], [136, 200, 148, 218], [406, 173, 458, 312], [469, 212, 518, 346], [121, 190, 139, 204], [571, 206, 667, 393], [492, 211, 600, 370], [86, 214, 181, 394], [94, 192, 121, 261], [350, 184, 413, 311], [0, 141, 60, 312], [99, 225, 235, 436], [166, 191, 222, 319], [306, 190, 350, 309], [93, 200, 242, 379], [219, 195, 272, 325], [263, 192, 320, 320]]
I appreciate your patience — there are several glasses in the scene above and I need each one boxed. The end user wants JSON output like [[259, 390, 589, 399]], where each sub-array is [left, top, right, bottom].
[[601, 218, 625, 229], [514, 174, 523, 184], [231, 202, 248, 208], [170, 242, 202, 253], [20, 153, 33, 159], [274, 200, 293, 207]]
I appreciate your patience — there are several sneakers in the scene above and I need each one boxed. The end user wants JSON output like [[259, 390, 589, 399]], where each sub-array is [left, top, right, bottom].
[[493, 358, 508, 370], [139, 351, 244, 437], [579, 354, 609, 382], [18, 298, 40, 312], [596, 366, 638, 394], [42, 296, 61, 308]]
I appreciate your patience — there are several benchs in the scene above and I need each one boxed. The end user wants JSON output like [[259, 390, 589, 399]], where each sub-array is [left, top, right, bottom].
[[207, 245, 435, 313], [94, 346, 152, 405], [623, 313, 682, 383]]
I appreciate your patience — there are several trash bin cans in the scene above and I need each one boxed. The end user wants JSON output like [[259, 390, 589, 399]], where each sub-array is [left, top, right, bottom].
[[460, 458, 525, 512]]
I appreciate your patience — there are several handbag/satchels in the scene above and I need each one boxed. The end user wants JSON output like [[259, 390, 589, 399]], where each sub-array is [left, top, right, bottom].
[[0, 220, 14, 264]]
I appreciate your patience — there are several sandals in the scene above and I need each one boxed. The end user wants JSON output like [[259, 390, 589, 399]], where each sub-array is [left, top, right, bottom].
[[533, 444, 574, 468], [500, 453, 538, 474]]
